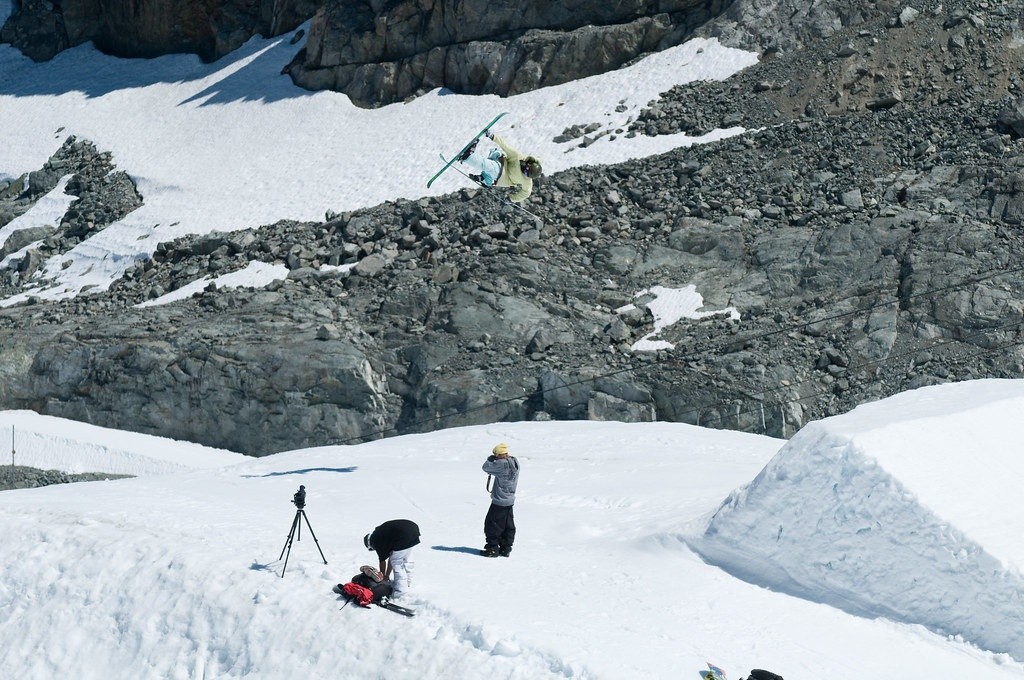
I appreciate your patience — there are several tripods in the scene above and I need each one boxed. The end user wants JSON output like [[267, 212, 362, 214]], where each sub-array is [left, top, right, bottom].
[[279, 504, 327, 578]]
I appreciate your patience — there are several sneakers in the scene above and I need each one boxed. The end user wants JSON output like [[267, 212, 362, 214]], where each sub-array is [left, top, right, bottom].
[[499, 548, 510, 557], [457, 138, 480, 164], [480, 548, 499, 557], [469, 173, 485, 182]]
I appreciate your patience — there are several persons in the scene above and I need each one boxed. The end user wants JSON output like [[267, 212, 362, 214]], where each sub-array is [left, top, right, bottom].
[[364, 518, 421, 600], [479, 444, 519, 557], [458, 131, 542, 205]]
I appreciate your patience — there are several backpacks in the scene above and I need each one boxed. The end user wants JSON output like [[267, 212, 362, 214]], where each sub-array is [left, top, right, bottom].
[[332, 565, 393, 610]]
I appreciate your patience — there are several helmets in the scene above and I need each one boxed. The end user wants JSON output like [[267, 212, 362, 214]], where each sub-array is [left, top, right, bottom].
[[528, 162, 542, 178]]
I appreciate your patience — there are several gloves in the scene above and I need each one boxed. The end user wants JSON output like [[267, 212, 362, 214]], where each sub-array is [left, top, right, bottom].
[[484, 129, 494, 141], [487, 455, 496, 462], [501, 196, 514, 205]]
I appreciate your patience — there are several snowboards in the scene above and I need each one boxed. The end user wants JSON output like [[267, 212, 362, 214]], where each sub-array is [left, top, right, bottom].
[[427, 110, 510, 190]]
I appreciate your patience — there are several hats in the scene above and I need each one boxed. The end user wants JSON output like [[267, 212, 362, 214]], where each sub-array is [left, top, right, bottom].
[[364, 533, 373, 551], [493, 443, 508, 457]]
